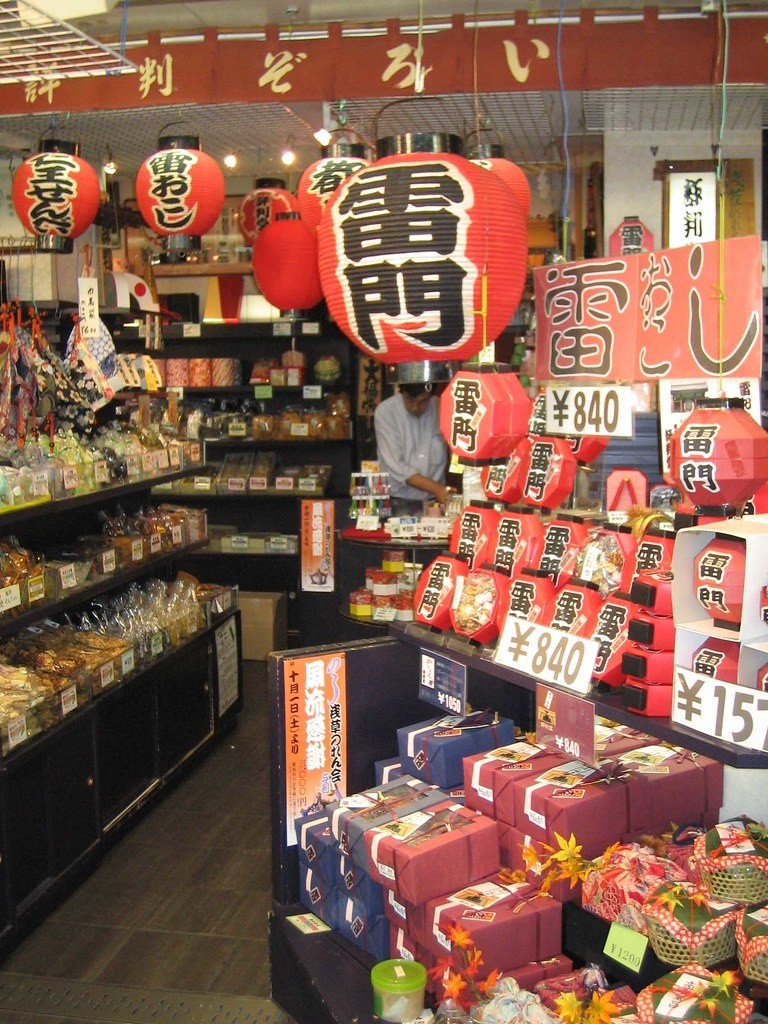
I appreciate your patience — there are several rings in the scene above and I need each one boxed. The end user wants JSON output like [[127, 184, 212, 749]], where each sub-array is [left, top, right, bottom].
[[445, 484, 451, 492]]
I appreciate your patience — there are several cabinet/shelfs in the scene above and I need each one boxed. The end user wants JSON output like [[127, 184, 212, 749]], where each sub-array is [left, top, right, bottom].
[[260, 622, 768, 1024], [111, 262, 354, 594], [0, 462, 244, 964], [336, 534, 450, 629]]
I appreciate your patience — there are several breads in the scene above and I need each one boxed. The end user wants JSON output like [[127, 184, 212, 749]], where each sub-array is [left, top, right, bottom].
[[253, 392, 353, 441], [0, 503, 231, 757]]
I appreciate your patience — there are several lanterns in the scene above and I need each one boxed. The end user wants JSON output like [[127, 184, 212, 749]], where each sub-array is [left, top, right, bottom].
[[440, 363, 533, 466], [693, 532, 745, 631], [239, 133, 532, 384], [136, 135, 226, 251], [670, 397, 768, 516], [13, 139, 101, 254]]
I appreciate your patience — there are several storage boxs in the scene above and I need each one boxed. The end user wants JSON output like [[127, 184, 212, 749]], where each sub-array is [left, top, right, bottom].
[[0, 358, 768, 1024]]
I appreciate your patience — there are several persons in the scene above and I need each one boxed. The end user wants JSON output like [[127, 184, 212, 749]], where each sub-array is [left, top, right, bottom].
[[374, 383, 458, 569]]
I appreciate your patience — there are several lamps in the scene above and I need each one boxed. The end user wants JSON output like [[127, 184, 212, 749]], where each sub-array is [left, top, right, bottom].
[[312, 101, 339, 148], [103, 142, 118, 174]]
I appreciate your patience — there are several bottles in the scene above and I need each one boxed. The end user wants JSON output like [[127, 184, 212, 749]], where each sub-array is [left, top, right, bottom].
[[371, 959, 428, 1021]]
